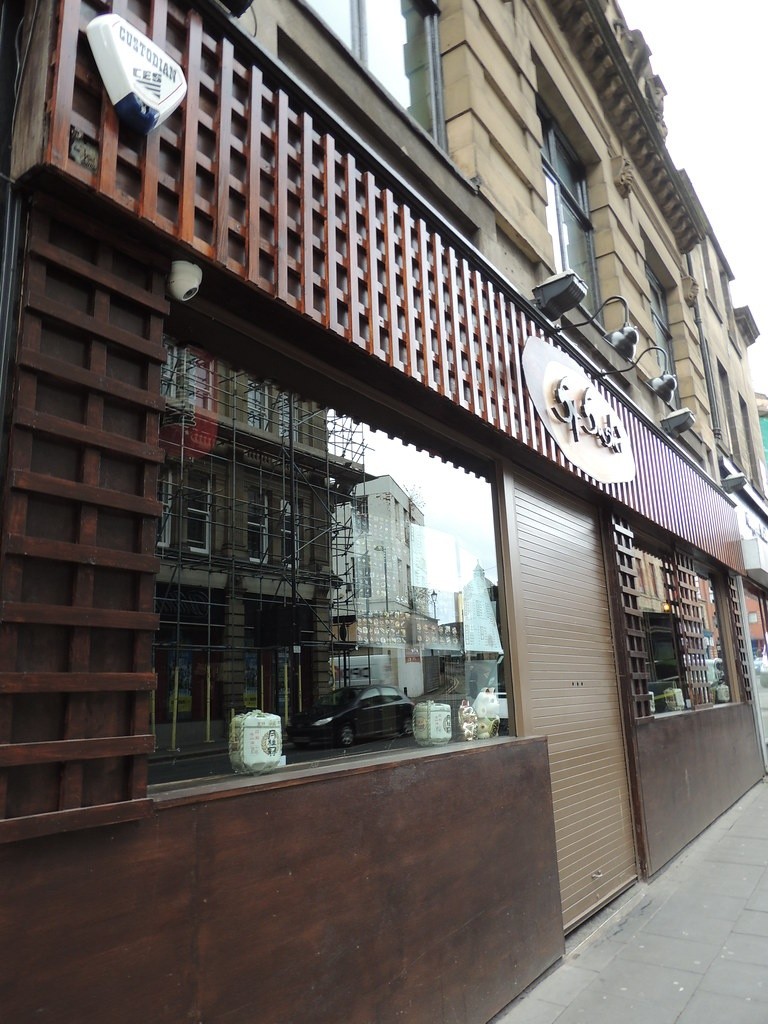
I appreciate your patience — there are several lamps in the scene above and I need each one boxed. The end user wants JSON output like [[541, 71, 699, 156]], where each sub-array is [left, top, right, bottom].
[[720, 472, 748, 494], [659, 407, 696, 439], [601, 346, 677, 406], [555, 296, 639, 365], [531, 268, 589, 322]]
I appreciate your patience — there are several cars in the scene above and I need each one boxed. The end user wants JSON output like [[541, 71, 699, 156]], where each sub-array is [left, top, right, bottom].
[[289, 684, 415, 749]]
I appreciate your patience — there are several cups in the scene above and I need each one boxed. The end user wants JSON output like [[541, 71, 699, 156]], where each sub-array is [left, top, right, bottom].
[[229, 710, 282, 771], [664, 688, 684, 710], [413, 700, 452, 747], [716, 685, 730, 703]]
